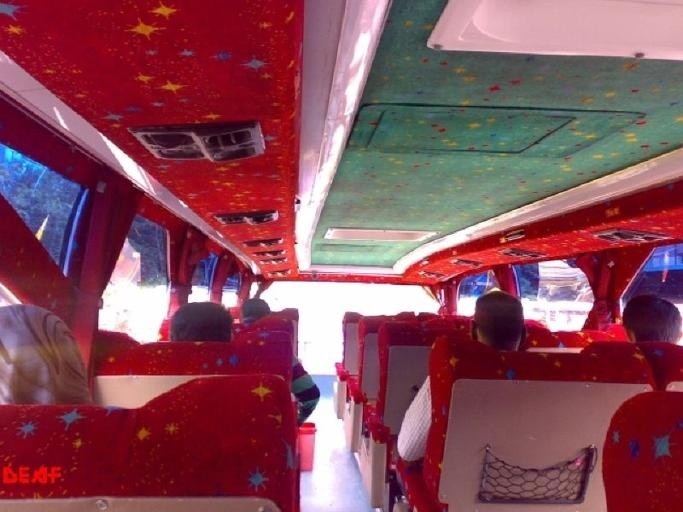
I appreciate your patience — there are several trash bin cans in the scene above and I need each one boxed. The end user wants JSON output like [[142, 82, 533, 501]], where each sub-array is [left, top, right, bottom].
[[298, 423, 316, 470]]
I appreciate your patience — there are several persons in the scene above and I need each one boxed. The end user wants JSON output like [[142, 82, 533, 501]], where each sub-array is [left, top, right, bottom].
[[395, 291, 527, 462], [622, 294, 682, 351], [239, 297, 321, 429], [0, 304, 98, 406], [169, 301, 235, 341]]
